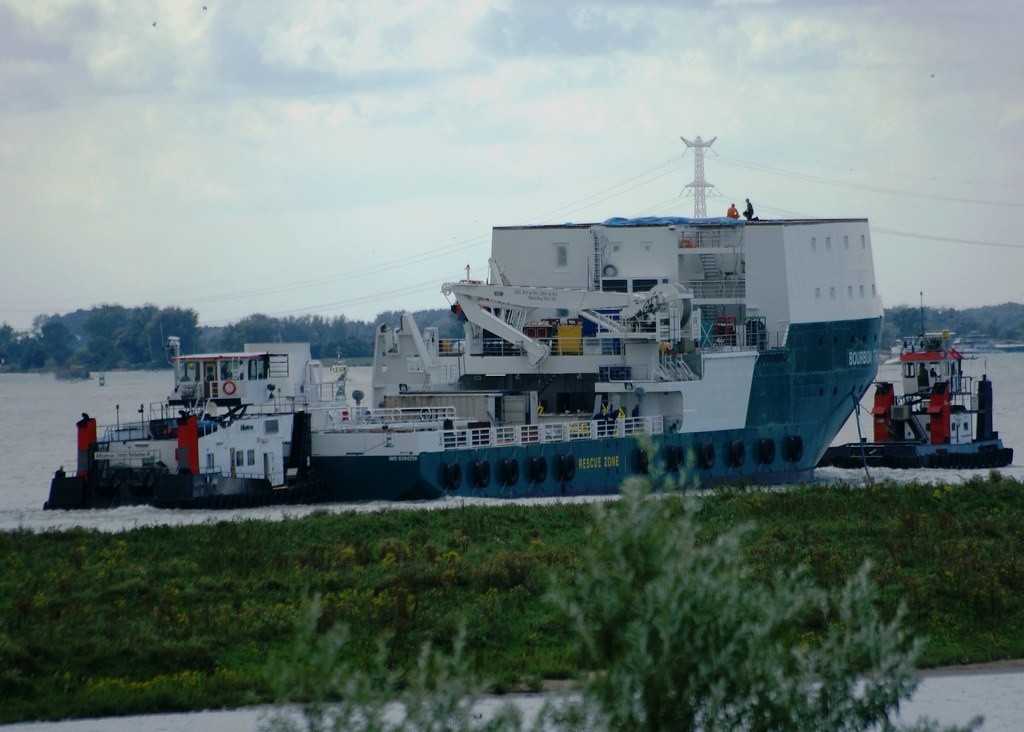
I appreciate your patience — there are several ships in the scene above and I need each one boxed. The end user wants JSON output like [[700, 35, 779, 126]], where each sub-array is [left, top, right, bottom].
[[43, 133, 885, 515]]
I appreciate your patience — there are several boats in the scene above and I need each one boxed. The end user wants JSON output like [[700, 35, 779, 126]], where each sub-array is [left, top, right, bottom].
[[815, 288, 1017, 469]]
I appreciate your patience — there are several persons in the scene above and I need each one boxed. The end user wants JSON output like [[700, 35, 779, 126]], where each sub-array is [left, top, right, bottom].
[[593, 405, 638, 436], [728, 198, 759, 222], [221, 361, 243, 380], [930, 367, 938, 378]]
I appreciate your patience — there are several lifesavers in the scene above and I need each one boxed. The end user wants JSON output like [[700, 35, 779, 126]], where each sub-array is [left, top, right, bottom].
[[630, 432, 806, 477], [220, 379, 238, 396], [438, 452, 579, 493]]
